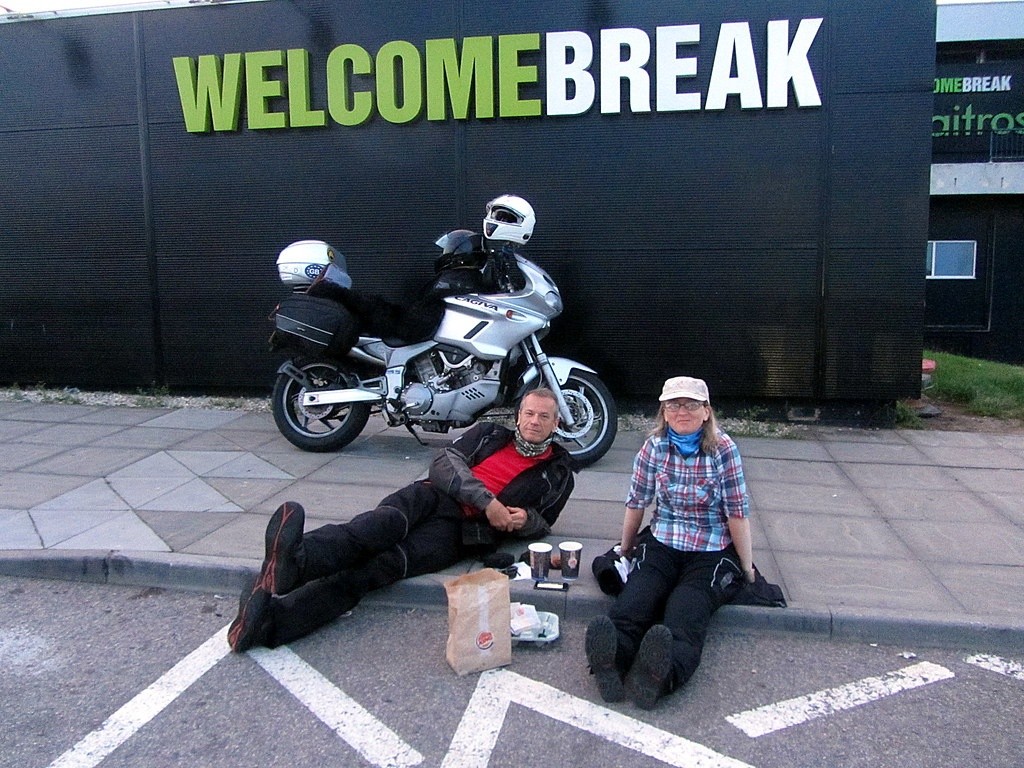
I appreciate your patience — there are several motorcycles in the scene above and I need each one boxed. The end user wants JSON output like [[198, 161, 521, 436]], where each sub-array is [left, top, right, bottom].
[[267, 211, 619, 466]]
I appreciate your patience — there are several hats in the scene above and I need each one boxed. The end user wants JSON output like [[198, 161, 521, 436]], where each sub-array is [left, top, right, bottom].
[[659, 376, 711, 404]]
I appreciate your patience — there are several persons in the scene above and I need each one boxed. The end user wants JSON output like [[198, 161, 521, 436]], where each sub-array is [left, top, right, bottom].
[[585, 376, 757, 708], [227, 387, 575, 653]]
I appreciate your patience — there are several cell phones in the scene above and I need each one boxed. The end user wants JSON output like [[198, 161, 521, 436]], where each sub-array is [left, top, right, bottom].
[[534, 580, 569, 591]]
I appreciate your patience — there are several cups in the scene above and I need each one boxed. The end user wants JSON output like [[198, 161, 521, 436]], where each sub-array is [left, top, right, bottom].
[[559, 542, 583, 580], [528, 543, 552, 581]]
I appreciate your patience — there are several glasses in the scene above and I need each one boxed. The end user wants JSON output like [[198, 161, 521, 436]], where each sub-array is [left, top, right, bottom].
[[663, 402, 704, 412]]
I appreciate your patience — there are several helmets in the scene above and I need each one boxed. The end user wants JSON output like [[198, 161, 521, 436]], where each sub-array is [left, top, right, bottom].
[[433, 229, 485, 273], [483, 194, 536, 245]]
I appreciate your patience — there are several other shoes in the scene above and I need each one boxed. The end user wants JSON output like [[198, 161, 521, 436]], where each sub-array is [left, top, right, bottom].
[[227, 573, 273, 653], [585, 615, 625, 703], [633, 624, 673, 711], [259, 501, 305, 594]]
